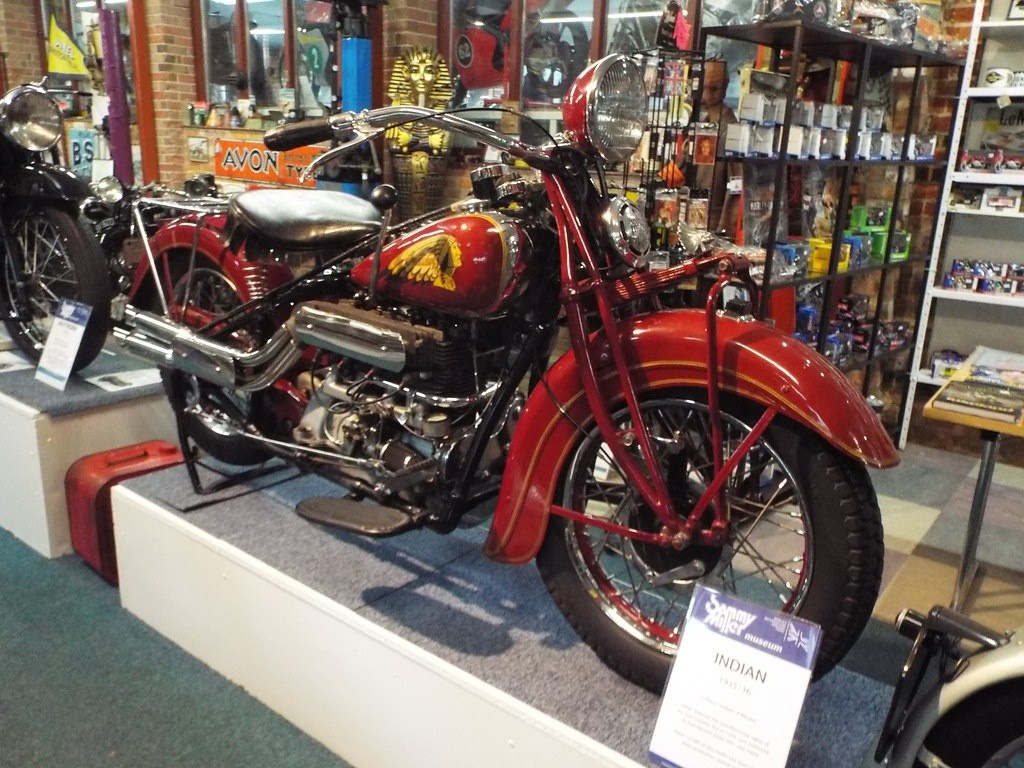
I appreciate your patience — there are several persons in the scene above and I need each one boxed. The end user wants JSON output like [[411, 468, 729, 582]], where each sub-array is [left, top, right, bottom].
[[385, 44, 455, 228], [688, 59, 735, 207]]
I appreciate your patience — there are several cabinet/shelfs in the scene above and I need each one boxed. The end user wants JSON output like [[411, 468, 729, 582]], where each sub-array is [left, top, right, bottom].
[[889, 0, 1024, 452], [682, 20, 964, 464]]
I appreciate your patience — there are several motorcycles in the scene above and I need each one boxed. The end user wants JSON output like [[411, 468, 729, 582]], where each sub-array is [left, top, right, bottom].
[[0, 75, 111, 377], [95, 53, 906, 705]]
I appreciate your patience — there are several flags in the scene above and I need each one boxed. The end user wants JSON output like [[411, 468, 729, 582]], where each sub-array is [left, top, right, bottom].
[[49, 13, 93, 82]]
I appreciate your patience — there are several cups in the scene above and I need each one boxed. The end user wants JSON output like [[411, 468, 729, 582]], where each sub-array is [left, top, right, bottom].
[[987, 67, 1015, 87]]
